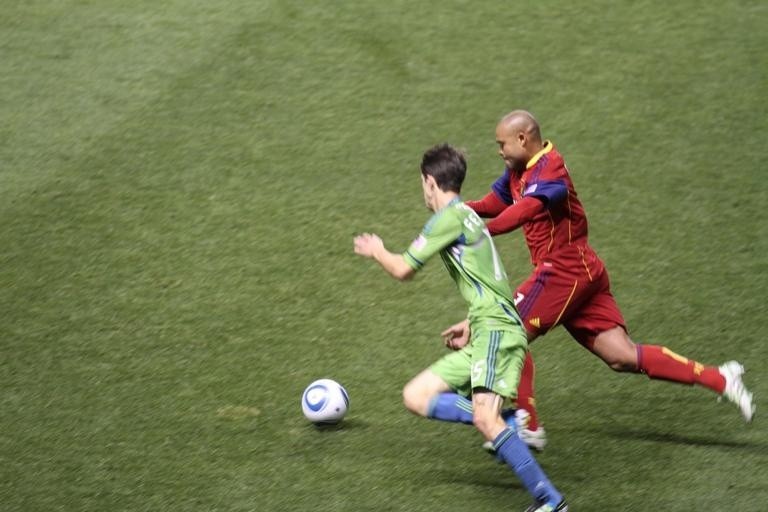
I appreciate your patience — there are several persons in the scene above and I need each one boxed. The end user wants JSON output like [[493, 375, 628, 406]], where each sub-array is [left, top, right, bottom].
[[352, 140, 571, 511], [464, 109, 758, 452]]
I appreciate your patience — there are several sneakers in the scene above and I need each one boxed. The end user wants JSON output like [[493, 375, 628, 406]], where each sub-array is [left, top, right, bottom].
[[523, 495, 569, 512], [482, 408, 549, 465], [717, 359, 757, 424]]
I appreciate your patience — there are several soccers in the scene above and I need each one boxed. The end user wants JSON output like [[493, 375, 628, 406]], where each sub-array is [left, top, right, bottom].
[[302, 378, 349, 425]]
